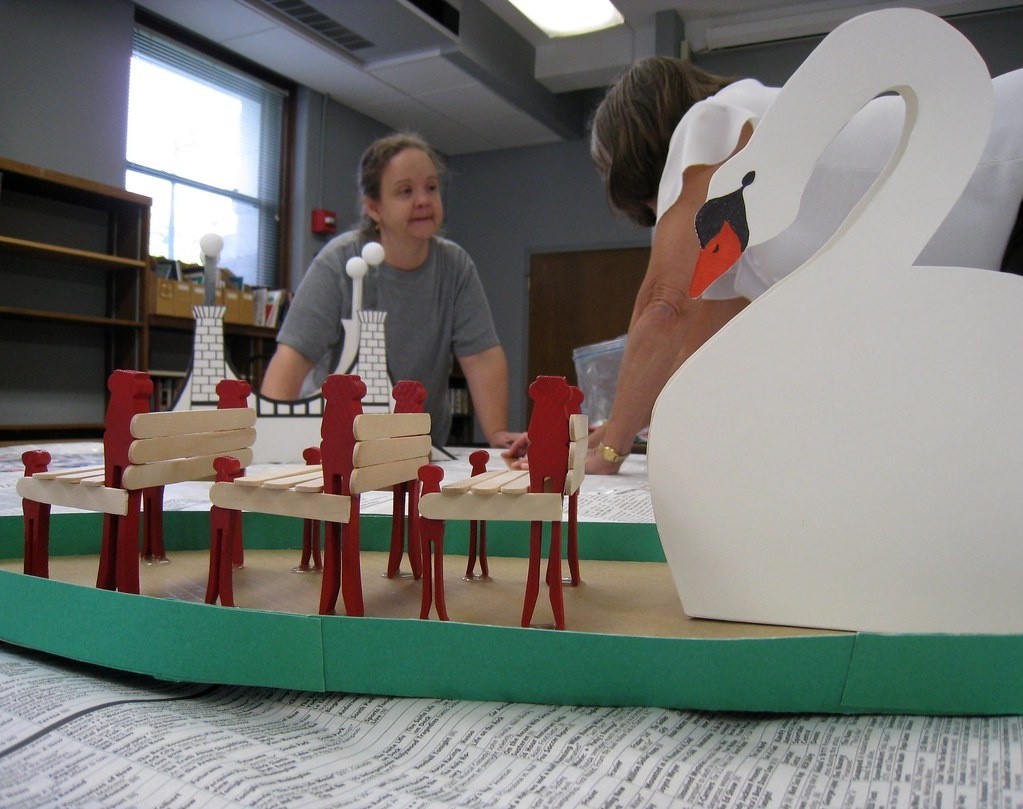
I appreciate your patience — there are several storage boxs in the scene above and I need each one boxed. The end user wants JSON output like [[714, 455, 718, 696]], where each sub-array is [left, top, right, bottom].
[[150, 255, 256, 327], [572, 335, 634, 429], [0, 509, 1023, 718]]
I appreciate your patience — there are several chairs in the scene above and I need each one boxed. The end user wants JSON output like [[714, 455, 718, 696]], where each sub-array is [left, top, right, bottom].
[[16, 368, 589, 631]]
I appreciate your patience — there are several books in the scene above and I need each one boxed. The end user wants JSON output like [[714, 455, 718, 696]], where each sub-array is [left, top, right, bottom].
[[449, 388, 468, 414], [255, 288, 287, 328], [154, 260, 205, 284], [216, 270, 243, 291]]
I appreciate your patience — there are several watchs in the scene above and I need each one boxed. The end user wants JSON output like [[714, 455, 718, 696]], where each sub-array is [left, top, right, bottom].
[[598, 440, 630, 462]]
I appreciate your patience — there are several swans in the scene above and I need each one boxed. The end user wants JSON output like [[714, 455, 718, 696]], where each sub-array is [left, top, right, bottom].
[[643, 7, 1022, 638]]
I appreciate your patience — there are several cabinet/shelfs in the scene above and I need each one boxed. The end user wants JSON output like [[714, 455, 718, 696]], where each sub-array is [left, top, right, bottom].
[[1, 155, 152, 447]]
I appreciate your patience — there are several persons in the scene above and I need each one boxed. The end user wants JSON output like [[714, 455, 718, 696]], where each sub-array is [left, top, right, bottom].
[[263, 135, 525, 449], [501, 58, 1022, 475]]
